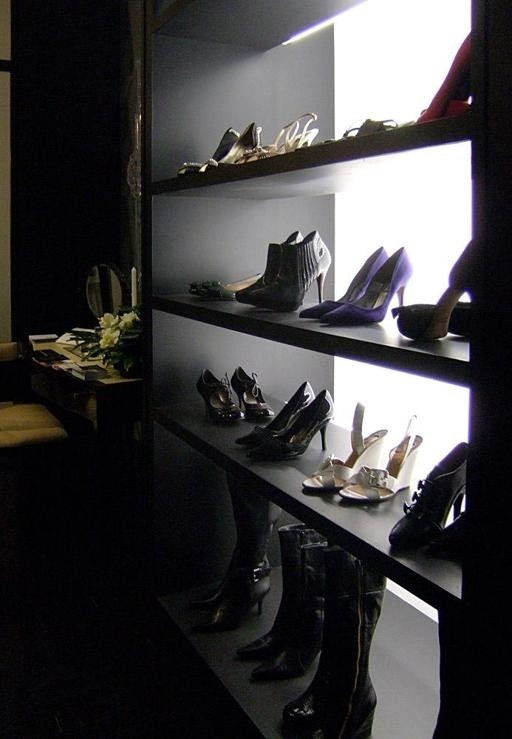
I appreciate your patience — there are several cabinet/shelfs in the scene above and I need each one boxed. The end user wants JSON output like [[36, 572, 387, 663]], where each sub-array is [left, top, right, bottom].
[[140, 0, 508, 739]]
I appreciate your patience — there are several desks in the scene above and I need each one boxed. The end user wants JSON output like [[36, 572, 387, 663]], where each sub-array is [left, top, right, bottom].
[[27, 354, 140, 486]]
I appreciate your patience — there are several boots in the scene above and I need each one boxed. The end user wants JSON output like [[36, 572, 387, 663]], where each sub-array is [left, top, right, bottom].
[[281, 536, 387, 739], [192, 474, 271, 631], [250, 540, 328, 686], [236, 524, 322, 661]]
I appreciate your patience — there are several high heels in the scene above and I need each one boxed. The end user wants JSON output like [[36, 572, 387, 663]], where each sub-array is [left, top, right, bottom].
[[236, 230, 471, 341], [229, 366, 274, 423], [339, 415, 423, 501], [196, 367, 245, 425], [235, 383, 332, 461], [301, 402, 389, 490], [388, 442, 464, 560]]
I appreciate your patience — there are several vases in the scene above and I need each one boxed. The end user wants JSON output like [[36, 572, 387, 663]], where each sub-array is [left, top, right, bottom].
[[113, 357, 141, 379]]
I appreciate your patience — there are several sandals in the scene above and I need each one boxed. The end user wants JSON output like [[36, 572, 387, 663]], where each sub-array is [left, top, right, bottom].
[[178, 113, 413, 174]]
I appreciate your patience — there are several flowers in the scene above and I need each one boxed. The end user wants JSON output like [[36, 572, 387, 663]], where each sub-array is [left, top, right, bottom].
[[72, 310, 140, 372]]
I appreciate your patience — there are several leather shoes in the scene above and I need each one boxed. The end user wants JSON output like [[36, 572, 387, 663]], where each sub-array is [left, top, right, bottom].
[[418, 33, 472, 123], [190, 273, 260, 300]]
